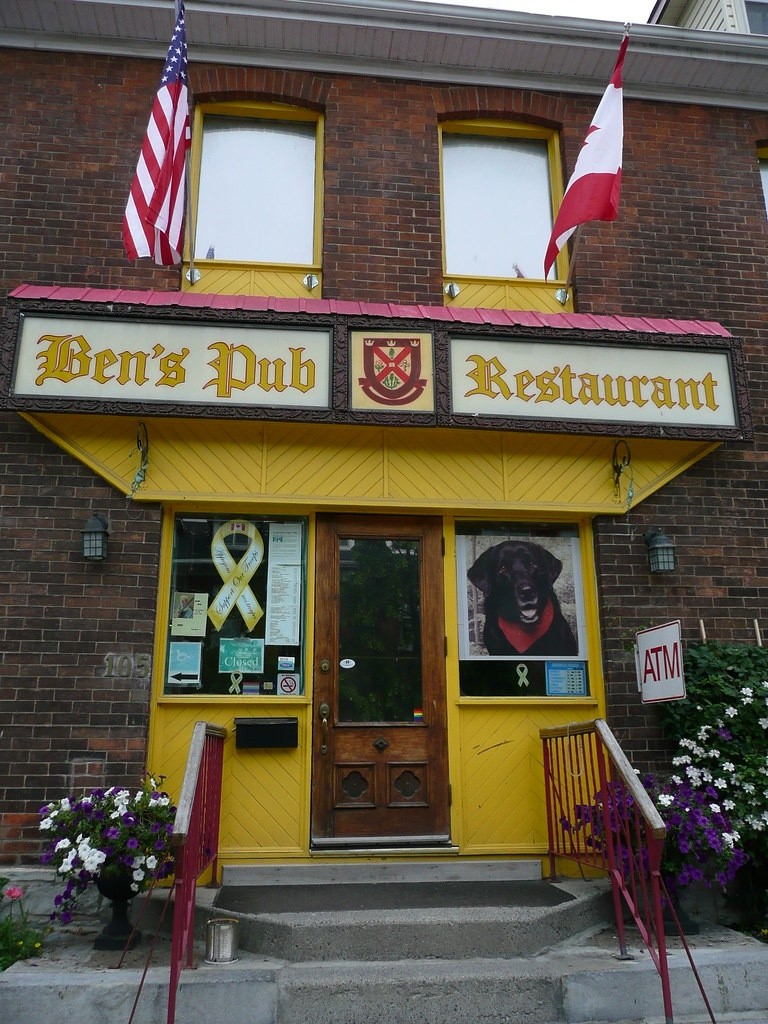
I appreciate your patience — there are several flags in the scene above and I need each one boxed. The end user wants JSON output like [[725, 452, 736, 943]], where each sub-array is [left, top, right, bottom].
[[121, 3, 191, 267], [543, 33, 630, 285]]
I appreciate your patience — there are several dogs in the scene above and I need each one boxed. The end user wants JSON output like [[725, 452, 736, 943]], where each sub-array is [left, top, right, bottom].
[[467, 541, 579, 656]]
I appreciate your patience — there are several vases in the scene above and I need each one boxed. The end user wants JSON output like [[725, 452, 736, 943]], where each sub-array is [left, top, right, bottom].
[[91, 872, 138, 951], [644, 847, 702, 935]]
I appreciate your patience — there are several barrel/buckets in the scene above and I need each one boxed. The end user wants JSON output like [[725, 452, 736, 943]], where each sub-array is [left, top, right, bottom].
[[206, 918, 239, 963]]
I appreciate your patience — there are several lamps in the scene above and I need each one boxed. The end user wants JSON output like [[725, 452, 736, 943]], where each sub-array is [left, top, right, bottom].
[[81, 513, 109, 560], [644, 529, 677, 573]]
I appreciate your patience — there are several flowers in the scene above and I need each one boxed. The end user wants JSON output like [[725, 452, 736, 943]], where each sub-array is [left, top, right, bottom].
[[558, 776, 749, 906], [37, 774, 179, 925]]
